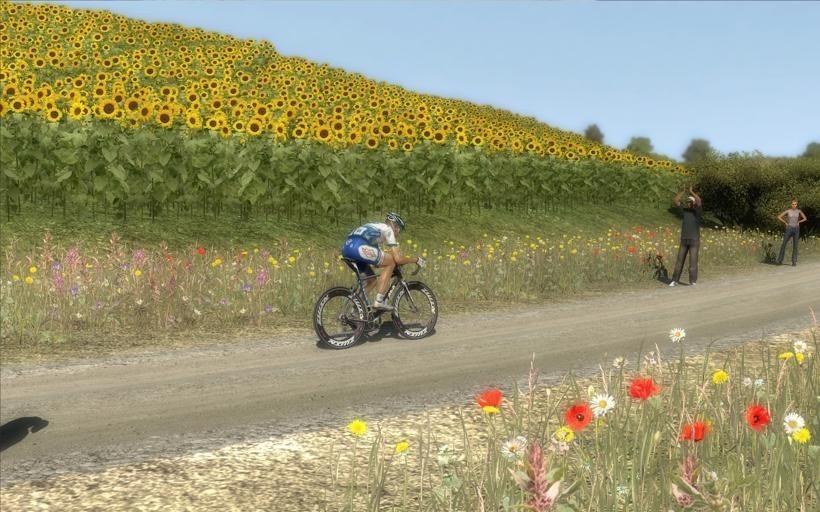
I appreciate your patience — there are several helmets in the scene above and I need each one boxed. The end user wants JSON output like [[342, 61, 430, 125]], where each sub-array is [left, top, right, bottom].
[[385, 212, 405, 230]]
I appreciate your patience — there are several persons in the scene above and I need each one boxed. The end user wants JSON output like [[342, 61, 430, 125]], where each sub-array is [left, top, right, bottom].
[[668, 183, 703, 287], [343, 210, 427, 313], [774, 198, 808, 265]]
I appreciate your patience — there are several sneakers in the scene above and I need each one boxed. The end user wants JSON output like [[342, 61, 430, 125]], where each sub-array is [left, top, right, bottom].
[[669, 281, 677, 287], [373, 301, 393, 311]]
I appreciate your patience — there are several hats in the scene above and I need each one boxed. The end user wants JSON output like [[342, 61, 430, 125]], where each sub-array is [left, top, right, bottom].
[[686, 197, 695, 202]]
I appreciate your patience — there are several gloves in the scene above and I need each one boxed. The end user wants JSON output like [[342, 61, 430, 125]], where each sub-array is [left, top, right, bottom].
[[415, 257, 423, 268]]
